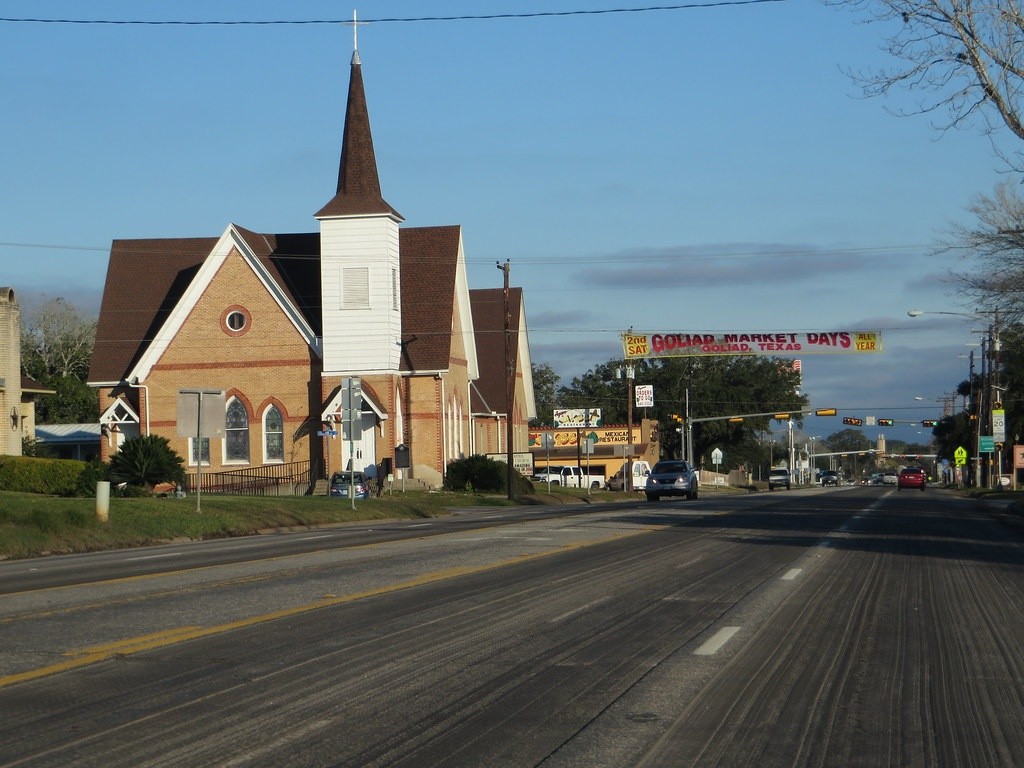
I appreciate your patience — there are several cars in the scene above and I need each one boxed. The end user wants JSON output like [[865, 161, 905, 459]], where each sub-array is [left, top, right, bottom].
[[898, 468, 925, 491], [330, 472, 370, 501], [821, 470, 838, 487], [882, 472, 899, 486]]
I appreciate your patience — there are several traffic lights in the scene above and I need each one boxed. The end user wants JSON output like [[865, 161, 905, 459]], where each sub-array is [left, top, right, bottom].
[[842, 417, 862, 426], [923, 420, 938, 427], [995, 443, 1003, 451], [993, 402, 1002, 409], [878, 419, 893, 426]]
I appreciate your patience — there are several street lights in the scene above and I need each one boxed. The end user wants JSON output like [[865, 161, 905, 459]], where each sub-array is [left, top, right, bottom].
[[907, 309, 1004, 490]]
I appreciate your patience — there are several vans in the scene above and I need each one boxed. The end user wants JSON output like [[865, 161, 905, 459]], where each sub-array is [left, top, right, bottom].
[[768, 465, 791, 491], [606, 460, 651, 492]]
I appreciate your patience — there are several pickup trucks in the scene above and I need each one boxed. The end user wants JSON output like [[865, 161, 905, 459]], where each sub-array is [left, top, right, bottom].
[[534, 464, 605, 490]]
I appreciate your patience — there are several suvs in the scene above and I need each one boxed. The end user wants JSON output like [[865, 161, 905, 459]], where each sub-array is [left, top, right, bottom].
[[645, 459, 699, 502]]
